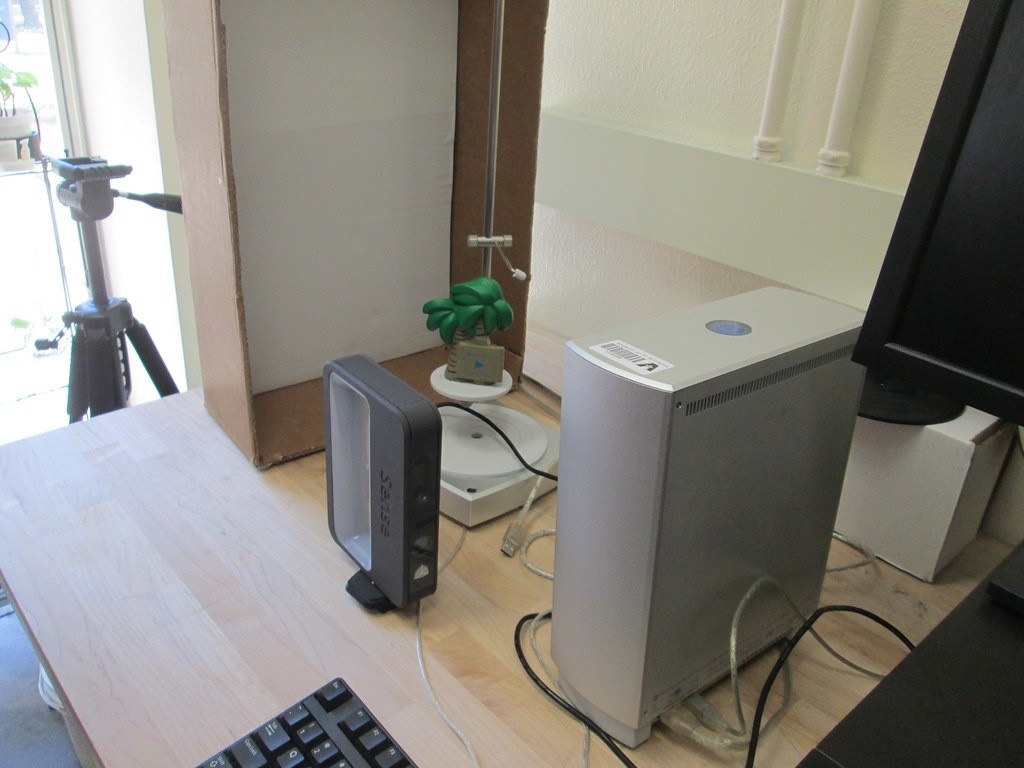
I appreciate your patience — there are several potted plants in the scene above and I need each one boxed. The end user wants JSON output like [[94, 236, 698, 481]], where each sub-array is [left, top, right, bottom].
[[0, 66, 38, 141]]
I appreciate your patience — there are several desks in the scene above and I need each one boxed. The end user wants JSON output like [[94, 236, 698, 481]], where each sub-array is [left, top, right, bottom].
[[0, 388, 1014, 768]]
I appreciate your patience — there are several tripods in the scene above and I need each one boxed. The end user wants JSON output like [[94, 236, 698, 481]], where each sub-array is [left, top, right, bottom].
[[34, 157, 182, 426]]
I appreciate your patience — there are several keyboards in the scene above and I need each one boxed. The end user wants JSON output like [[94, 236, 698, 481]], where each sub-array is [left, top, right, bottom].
[[196, 677, 420, 768]]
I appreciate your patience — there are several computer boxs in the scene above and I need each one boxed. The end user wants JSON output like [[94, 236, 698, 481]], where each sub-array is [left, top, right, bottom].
[[549, 288, 871, 751]]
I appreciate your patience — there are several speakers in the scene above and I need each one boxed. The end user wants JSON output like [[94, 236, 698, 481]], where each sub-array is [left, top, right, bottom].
[[834, 405, 1017, 583], [322, 355, 442, 613]]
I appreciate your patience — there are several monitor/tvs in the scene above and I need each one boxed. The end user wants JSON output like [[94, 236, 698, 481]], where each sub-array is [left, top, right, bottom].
[[849, 0, 1024, 425]]
